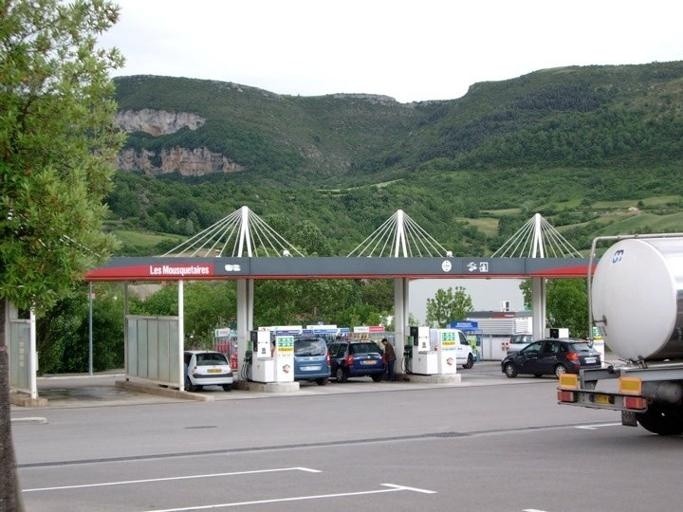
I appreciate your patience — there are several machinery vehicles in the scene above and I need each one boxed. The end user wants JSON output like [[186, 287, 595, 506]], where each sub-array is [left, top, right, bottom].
[[557, 233, 683, 434]]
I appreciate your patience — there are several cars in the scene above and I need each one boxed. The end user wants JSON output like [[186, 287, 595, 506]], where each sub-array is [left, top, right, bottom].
[[184, 350, 233, 391], [294, 336, 388, 385], [501, 337, 601, 379]]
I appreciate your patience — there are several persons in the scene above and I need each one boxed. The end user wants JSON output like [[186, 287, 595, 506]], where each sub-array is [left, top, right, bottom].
[[381, 339, 396, 378]]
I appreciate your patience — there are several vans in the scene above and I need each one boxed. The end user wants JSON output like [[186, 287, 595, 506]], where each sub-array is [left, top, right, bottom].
[[457, 330, 477, 370], [506, 332, 532, 357]]
